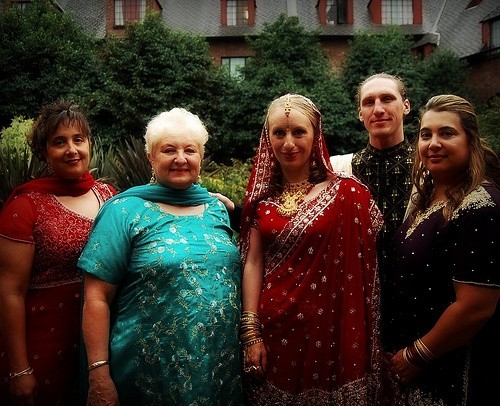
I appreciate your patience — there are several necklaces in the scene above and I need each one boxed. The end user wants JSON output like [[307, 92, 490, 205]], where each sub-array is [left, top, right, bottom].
[[277, 179, 315, 217]]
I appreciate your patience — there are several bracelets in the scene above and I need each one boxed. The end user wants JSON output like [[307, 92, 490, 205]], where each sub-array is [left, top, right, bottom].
[[240, 311, 264, 348], [403, 338, 435, 369], [87, 360, 109, 372], [9, 366, 33, 379]]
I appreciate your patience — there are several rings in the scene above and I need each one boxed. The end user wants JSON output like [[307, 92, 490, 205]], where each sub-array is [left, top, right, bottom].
[[253, 366, 260, 371], [244, 366, 253, 372], [393, 373, 400, 384]]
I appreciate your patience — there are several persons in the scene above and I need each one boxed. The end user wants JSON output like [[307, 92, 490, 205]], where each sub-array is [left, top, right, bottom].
[[327, 73, 423, 240], [386, 94, 500, 405], [78, 108, 243, 405], [0, 103, 119, 406], [241, 93, 384, 404]]
